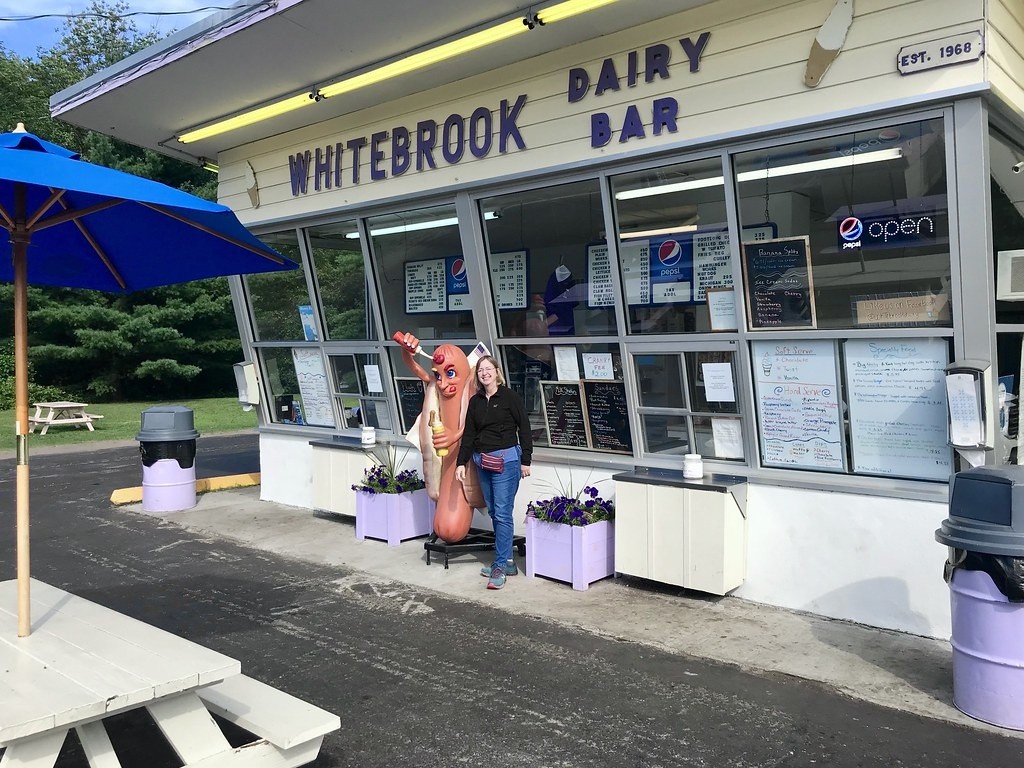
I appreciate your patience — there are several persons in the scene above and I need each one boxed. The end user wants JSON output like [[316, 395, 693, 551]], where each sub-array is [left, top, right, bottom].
[[456, 355, 533, 590]]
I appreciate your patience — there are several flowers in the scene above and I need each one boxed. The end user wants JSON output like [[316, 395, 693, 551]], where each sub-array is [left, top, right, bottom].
[[524, 452, 616, 534], [350, 430, 426, 496]]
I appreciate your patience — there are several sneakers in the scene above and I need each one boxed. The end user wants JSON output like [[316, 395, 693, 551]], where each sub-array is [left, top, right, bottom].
[[480, 560, 518, 576], [486, 564, 507, 589]]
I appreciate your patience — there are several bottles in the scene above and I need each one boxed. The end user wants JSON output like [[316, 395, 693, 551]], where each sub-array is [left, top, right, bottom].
[[362, 428, 375, 444], [684, 454, 703, 480]]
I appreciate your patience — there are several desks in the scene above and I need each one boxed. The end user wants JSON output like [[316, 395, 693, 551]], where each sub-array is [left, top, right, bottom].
[[28, 401, 100, 436], [0, 575, 325, 768]]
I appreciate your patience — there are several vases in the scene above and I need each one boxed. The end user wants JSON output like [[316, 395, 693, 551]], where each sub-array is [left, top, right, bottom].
[[356, 487, 436, 547], [525, 517, 616, 591]]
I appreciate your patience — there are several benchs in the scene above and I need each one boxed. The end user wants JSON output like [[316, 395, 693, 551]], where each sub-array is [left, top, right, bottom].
[[28, 416, 50, 422], [192, 672, 341, 751], [73, 413, 105, 418]]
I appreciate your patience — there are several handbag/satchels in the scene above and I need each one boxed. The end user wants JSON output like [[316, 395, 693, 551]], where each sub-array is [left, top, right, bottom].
[[479, 452, 503, 474]]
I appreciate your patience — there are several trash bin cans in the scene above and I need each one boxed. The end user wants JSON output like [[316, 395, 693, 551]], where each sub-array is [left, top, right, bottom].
[[935, 462, 1024, 738], [135, 406, 201, 512]]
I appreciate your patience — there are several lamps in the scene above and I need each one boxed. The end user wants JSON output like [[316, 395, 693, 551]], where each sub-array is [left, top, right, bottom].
[[615, 139, 912, 201], [529, 0, 619, 28], [342, 206, 504, 240], [175, 86, 321, 144], [313, 6, 537, 101]]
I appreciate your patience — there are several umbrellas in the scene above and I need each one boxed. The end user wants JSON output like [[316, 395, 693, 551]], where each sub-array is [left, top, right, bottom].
[[0, 125, 302, 637]]
